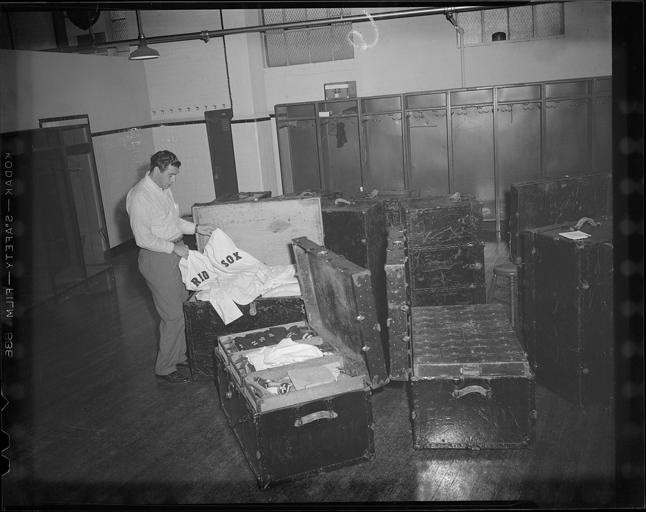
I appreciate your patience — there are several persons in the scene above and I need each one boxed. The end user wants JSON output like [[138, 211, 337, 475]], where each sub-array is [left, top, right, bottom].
[[124, 149, 220, 383]]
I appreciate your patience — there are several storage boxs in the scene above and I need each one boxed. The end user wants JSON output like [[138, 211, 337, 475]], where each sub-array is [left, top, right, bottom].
[[213, 237, 389, 492], [385, 247, 536, 449], [184, 197, 331, 383]]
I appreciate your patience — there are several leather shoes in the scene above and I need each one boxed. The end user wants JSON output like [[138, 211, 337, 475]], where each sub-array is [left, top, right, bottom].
[[155, 370, 190, 384]]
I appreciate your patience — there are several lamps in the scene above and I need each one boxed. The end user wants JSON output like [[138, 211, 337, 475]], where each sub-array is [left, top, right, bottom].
[[129, 10, 160, 60]]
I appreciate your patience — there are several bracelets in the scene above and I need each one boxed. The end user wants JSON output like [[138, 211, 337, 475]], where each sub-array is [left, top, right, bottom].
[[195, 223, 198, 233]]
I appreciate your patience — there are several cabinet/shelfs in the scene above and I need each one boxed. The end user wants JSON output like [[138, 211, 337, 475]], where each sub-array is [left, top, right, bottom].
[[524, 226, 614, 408], [399, 194, 487, 304], [321, 202, 383, 274]]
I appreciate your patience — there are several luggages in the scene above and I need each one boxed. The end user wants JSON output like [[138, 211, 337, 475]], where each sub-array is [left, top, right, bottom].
[[179, 191, 386, 491], [401, 193, 533, 450], [507, 171, 614, 405]]
